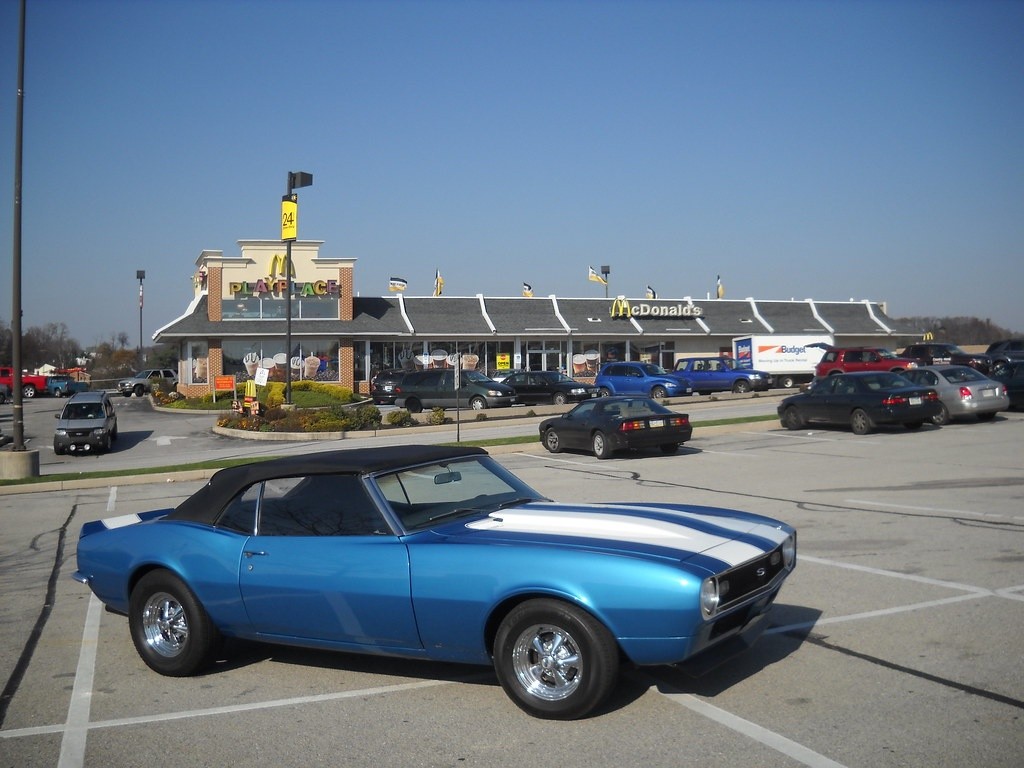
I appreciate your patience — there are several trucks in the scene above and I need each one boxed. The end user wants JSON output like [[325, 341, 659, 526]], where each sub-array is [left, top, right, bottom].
[[732, 335, 836, 388]]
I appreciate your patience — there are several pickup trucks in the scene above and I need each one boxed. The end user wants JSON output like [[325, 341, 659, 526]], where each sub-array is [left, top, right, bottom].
[[46, 375, 89, 398], [0, 366, 49, 399]]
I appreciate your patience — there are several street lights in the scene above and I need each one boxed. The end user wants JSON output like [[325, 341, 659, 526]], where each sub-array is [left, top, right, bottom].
[[600, 265, 611, 298], [284, 170, 315, 405], [136, 270, 145, 372]]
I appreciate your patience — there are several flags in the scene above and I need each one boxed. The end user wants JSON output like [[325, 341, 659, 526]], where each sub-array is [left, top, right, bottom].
[[522, 283, 534, 298], [646, 286, 658, 301], [434, 272, 444, 296], [389, 278, 407, 291], [588, 265, 608, 286], [717, 276, 724, 298]]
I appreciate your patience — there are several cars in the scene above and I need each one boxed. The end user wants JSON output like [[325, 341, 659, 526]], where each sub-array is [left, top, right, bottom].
[[499, 371, 599, 406], [538, 395, 693, 459], [890, 363, 1011, 426], [777, 370, 944, 436], [492, 370, 535, 384], [0, 384, 12, 405]]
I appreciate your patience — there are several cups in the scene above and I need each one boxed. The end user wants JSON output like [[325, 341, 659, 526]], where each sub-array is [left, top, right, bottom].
[[291, 357, 304, 376], [305, 356, 320, 378], [573, 354, 586, 373], [413, 354, 433, 371], [273, 353, 286, 369], [243, 353, 259, 376], [462, 353, 479, 369], [398, 350, 414, 369], [446, 353, 463, 369], [431, 349, 448, 369], [258, 357, 275, 377], [584, 349, 599, 372]]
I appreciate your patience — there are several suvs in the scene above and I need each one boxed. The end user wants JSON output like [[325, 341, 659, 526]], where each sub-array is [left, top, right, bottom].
[[984, 338, 1024, 411], [394, 368, 518, 414], [372, 368, 423, 405], [51, 390, 118, 455], [897, 343, 992, 375], [670, 356, 772, 395], [593, 362, 693, 399], [804, 341, 926, 378], [116, 367, 178, 397]]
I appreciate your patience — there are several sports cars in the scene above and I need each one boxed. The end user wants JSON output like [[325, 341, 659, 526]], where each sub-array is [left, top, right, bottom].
[[71, 443, 800, 720]]
